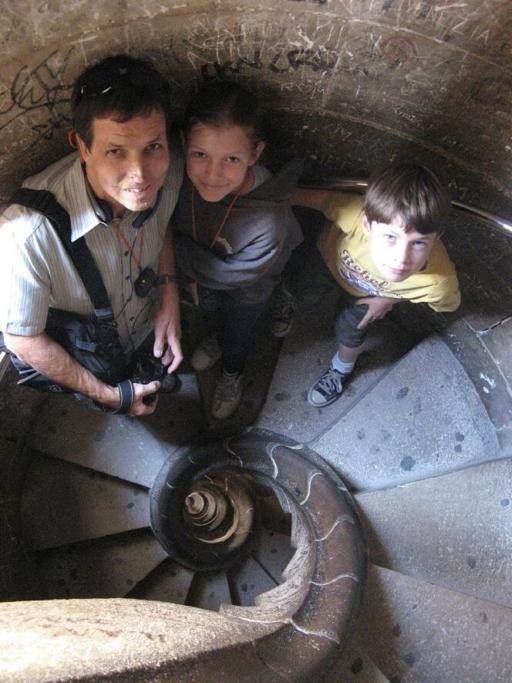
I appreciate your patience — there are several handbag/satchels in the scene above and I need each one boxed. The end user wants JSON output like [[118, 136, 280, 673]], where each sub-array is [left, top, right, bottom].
[[0, 307, 127, 394]]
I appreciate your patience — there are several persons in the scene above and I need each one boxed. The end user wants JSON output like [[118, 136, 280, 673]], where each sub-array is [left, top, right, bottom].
[[166, 73, 303, 427], [0, 50, 189, 430], [238, 150, 463, 410]]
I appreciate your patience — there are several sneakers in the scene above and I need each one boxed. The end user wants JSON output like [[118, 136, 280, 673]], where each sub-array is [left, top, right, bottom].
[[209, 370, 245, 420], [269, 288, 294, 338], [190, 331, 222, 372], [307, 364, 352, 407]]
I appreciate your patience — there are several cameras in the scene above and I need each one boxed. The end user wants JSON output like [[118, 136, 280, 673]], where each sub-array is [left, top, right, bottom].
[[129, 353, 182, 395]]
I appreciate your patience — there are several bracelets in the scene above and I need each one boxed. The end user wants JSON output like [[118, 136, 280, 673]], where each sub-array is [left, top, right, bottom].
[[157, 273, 175, 284]]
